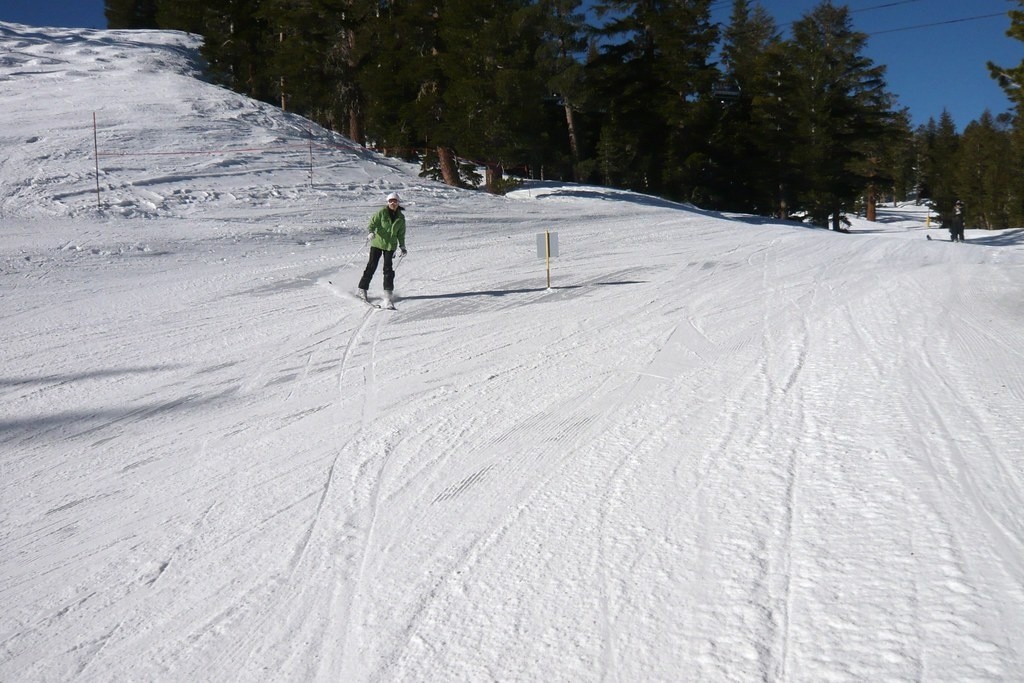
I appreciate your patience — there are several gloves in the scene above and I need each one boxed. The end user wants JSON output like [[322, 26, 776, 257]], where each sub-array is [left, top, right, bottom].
[[400, 247, 407, 257], [367, 233, 375, 240]]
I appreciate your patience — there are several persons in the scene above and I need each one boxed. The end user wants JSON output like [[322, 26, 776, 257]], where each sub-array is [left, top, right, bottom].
[[357, 193, 406, 310], [949, 214, 966, 242]]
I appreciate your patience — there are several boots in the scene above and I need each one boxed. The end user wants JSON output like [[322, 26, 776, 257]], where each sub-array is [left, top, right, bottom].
[[358, 288, 367, 300], [384, 290, 394, 309]]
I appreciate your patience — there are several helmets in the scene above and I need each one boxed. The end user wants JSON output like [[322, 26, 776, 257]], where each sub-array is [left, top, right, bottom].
[[387, 193, 399, 200]]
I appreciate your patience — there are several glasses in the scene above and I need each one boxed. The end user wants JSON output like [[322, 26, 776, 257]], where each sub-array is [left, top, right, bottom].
[[388, 199, 397, 203]]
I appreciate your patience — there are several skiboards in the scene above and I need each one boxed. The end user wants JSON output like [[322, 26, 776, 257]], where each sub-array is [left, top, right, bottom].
[[352, 295, 399, 310]]
